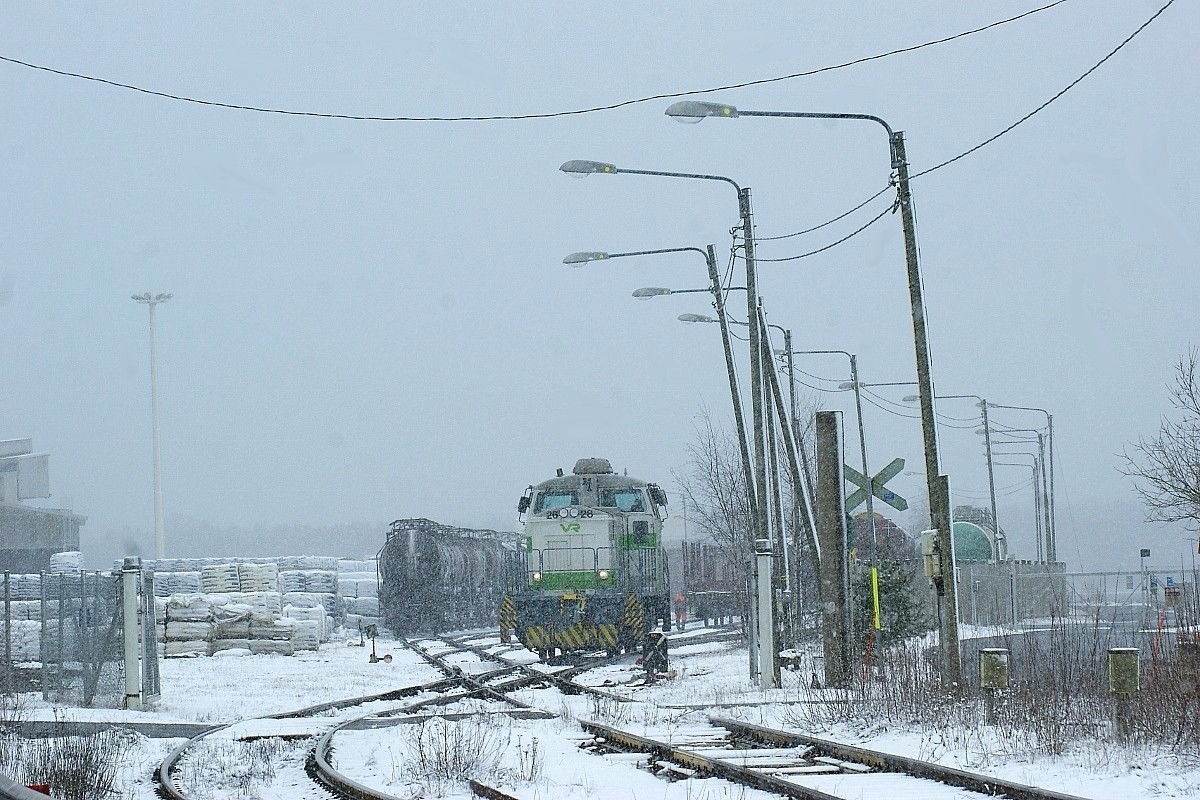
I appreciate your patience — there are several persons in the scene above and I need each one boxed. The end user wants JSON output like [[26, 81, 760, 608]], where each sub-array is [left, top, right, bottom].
[[675, 590, 686, 630]]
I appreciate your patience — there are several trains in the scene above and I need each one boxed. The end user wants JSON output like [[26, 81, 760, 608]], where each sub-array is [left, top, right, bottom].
[[515, 459, 668, 655]]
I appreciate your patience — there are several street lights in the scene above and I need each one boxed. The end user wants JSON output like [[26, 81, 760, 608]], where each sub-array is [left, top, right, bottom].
[[665, 100, 960, 694], [768, 347, 889, 681], [902, 392, 1002, 562], [132, 290, 173, 560], [974, 401, 1056, 561], [557, 158, 847, 687]]
